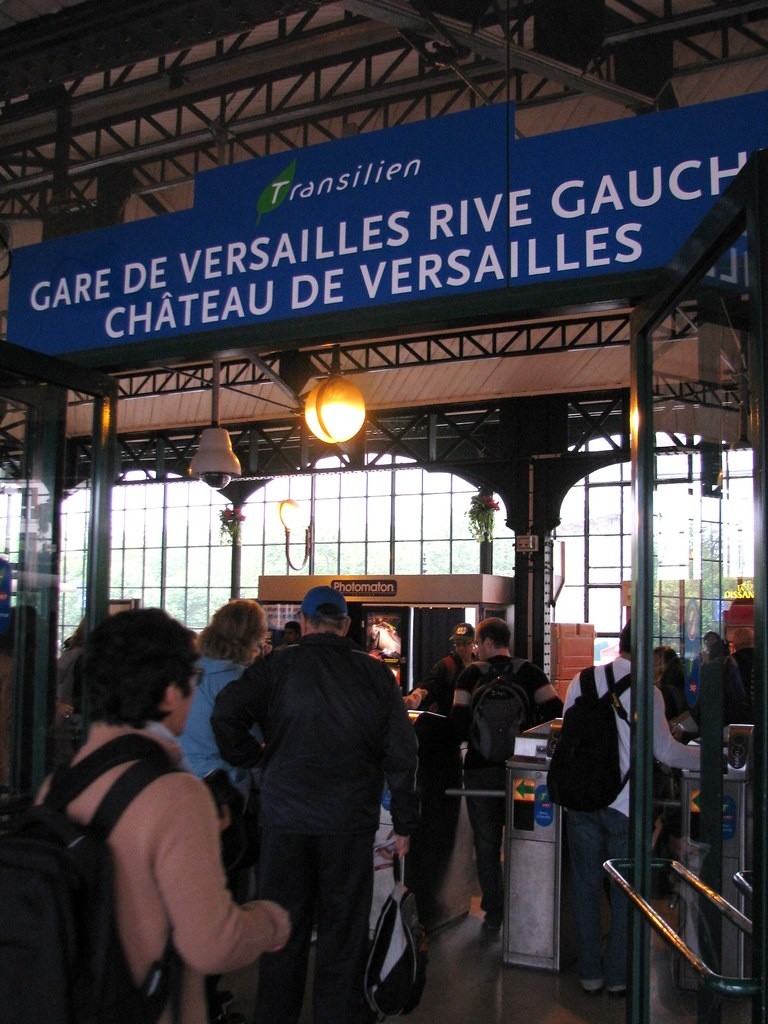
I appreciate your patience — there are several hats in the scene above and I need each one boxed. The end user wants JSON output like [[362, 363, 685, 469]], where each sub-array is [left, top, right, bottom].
[[449, 623, 474, 643], [295, 585, 347, 620]]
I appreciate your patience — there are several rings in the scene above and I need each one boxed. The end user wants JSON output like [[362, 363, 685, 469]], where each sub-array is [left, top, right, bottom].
[[65, 714, 70, 719]]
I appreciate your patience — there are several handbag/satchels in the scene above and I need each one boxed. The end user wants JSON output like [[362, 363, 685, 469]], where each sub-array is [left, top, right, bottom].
[[667, 710, 700, 745]]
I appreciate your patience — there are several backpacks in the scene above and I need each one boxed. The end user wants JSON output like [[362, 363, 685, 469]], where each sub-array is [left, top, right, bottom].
[[546, 666, 632, 809], [470, 657, 531, 766], [365, 853, 426, 1018], [0, 732, 184, 1024]]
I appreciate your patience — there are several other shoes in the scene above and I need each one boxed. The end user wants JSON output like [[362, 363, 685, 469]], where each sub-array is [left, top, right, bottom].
[[581, 962, 604, 990], [607, 984, 627, 991], [481, 921, 503, 937]]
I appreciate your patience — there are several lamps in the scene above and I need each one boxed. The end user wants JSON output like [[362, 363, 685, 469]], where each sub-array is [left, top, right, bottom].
[[188, 359, 242, 489], [304, 343, 366, 441], [280, 498, 312, 570]]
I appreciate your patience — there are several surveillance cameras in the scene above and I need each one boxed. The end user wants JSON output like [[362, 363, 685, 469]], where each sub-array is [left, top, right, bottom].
[[187, 428, 241, 489]]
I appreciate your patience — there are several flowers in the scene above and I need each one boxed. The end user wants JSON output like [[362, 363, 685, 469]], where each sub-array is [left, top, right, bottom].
[[468, 496, 500, 542], [220, 506, 244, 547]]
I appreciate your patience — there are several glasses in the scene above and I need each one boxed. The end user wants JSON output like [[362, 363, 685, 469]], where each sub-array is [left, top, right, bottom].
[[185, 668, 206, 687], [453, 641, 473, 648]]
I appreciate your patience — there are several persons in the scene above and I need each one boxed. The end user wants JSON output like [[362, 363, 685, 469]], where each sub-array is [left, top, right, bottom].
[[56, 607, 112, 719], [690, 630, 748, 723], [651, 644, 692, 858], [731, 626, 754, 705], [174, 598, 267, 1005], [367, 649, 384, 660], [34, 606, 293, 1024], [404, 621, 478, 716], [210, 585, 421, 1023], [446, 618, 564, 927], [282, 620, 300, 644], [562, 620, 700, 992]]
